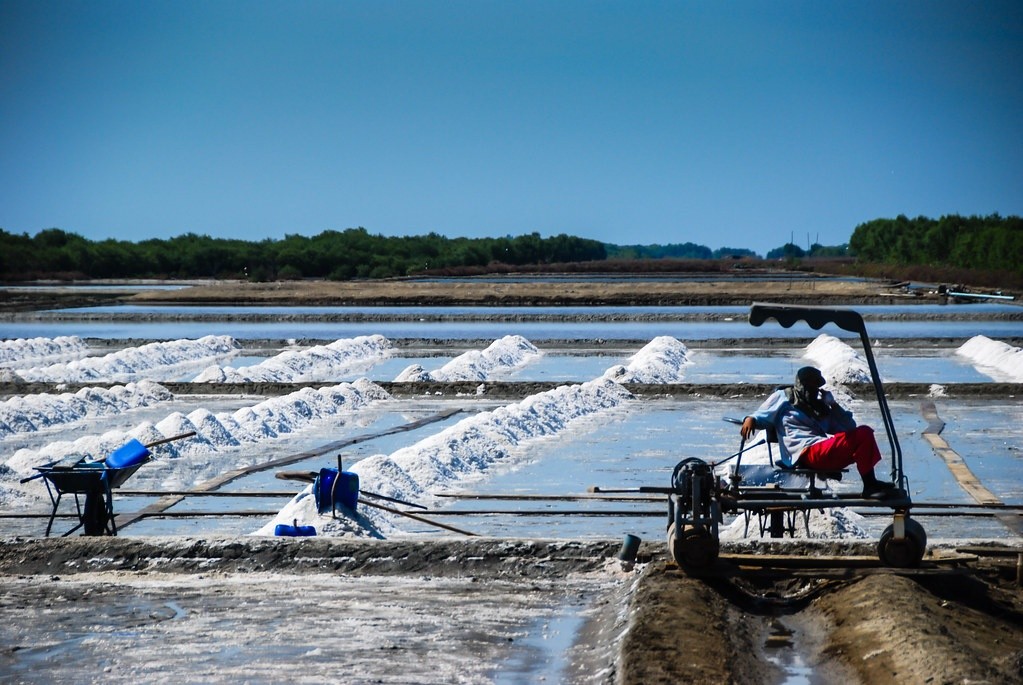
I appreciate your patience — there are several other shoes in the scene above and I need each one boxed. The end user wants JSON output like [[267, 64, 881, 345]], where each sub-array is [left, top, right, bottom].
[[862, 478, 895, 497]]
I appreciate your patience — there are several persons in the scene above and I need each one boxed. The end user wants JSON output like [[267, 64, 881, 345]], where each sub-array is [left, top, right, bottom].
[[740, 366, 899, 501]]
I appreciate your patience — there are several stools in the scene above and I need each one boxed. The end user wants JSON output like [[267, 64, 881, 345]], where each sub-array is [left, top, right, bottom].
[[776, 459, 850, 496]]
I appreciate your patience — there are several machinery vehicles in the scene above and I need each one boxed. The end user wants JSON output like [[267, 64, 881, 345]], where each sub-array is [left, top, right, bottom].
[[587, 297, 928, 574]]
